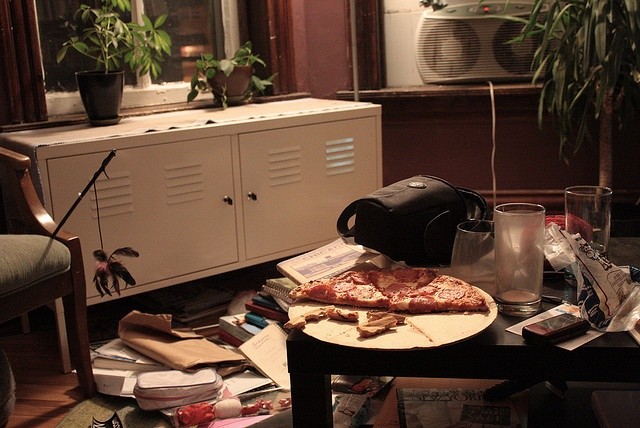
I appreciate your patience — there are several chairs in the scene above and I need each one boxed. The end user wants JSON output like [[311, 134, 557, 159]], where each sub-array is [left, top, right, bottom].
[[0, 146, 98, 398]]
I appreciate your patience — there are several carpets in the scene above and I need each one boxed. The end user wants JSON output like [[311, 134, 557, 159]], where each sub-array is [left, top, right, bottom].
[[54, 393, 171, 428]]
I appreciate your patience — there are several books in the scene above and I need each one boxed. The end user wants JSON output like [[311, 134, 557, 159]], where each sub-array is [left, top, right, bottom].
[[238, 323, 290, 392], [244, 236, 365, 328], [90, 337, 171, 371], [218, 309, 278, 348]]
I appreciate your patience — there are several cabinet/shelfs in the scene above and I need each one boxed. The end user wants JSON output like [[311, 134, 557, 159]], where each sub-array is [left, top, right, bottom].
[[346, 1, 640, 209], [0, 97, 384, 370]]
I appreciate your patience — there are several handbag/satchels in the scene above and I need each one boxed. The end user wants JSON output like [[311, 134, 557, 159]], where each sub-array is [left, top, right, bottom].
[[133, 367, 224, 411], [334, 175, 488, 267]]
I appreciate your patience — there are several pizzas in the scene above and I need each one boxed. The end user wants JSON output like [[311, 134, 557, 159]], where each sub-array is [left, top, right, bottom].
[[288, 268, 489, 315]]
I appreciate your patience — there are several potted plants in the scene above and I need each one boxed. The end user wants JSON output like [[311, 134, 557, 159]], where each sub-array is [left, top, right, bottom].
[[55, 0, 173, 125], [187, 40, 278, 109]]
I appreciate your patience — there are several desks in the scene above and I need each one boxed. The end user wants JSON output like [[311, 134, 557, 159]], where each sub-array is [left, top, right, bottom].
[[286, 237, 639, 424]]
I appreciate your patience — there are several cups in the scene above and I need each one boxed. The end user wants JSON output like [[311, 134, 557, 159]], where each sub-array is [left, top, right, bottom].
[[491, 201, 546, 319], [446, 219, 498, 298], [564, 185, 613, 259]]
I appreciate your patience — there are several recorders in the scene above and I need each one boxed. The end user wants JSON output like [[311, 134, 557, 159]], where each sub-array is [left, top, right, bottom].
[[412, 1, 552, 84]]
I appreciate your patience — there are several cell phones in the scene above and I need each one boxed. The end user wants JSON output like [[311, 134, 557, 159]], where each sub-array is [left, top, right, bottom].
[[522, 312, 592, 348]]
